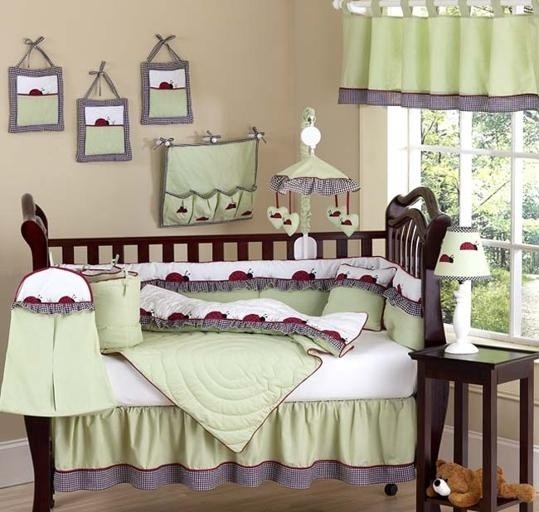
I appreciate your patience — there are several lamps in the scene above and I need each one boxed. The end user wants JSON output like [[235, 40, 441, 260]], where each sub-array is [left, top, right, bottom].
[[433, 226, 491, 354]]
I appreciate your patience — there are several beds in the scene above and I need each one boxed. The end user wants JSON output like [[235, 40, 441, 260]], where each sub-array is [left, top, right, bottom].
[[20, 186, 451, 512]]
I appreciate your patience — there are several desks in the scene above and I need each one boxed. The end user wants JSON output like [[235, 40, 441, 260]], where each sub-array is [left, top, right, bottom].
[[407, 343, 539, 512]]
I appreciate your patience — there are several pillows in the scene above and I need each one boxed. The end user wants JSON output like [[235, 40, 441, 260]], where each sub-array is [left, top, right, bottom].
[[320, 262, 396, 333]]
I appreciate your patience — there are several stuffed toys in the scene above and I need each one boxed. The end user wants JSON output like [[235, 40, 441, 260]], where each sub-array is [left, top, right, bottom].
[[425, 459, 538, 508]]
